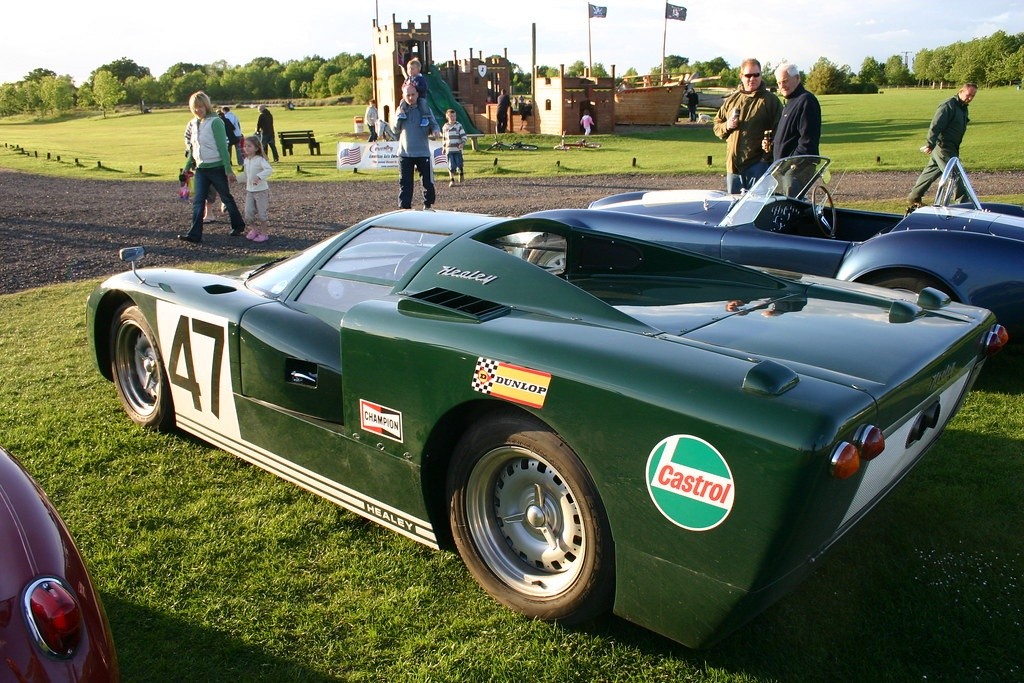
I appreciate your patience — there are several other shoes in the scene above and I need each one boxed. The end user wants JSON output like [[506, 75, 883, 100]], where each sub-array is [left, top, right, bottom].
[[460, 178, 464, 185], [423, 207, 433, 211], [247, 229, 258, 239], [220, 202, 228, 214], [253, 235, 269, 242], [230, 229, 241, 235], [177, 235, 200, 243], [906, 199, 927, 207], [204, 216, 215, 223], [449, 181, 455, 187]]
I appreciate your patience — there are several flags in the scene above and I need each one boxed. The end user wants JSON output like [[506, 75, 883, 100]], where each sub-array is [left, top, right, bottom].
[[588, 4, 607, 18], [666, 3, 687, 21]]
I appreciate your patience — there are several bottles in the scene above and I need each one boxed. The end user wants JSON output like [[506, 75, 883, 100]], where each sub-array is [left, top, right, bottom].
[[178, 174, 189, 199]]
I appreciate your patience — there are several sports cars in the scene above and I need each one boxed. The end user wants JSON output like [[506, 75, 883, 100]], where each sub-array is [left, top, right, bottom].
[[84, 206, 1010, 655], [521, 155, 1024, 379]]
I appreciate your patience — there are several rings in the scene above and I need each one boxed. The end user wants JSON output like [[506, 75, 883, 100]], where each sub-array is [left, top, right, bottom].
[[233, 175, 234, 177]]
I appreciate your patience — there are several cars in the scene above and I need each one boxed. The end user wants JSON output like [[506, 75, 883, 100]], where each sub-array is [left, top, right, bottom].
[[0, 443, 124, 683]]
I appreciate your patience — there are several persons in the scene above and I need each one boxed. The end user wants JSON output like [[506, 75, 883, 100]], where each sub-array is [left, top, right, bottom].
[[761, 62, 821, 199], [442, 109, 467, 187], [519, 95, 524, 102], [228, 135, 273, 241], [514, 97, 518, 108], [496, 88, 511, 133], [216, 106, 247, 171], [908, 83, 978, 205], [185, 117, 227, 223], [288, 101, 295, 110], [580, 109, 594, 136], [396, 58, 431, 126], [365, 99, 378, 142], [712, 58, 784, 195], [390, 85, 441, 211], [257, 104, 279, 162], [725, 297, 808, 317], [686, 88, 699, 121], [178, 90, 246, 242]]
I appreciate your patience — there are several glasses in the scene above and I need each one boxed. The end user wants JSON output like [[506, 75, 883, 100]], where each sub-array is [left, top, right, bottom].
[[744, 73, 759, 78]]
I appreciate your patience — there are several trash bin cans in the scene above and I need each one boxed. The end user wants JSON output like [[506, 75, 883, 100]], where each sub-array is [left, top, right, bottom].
[[354, 117, 363, 134]]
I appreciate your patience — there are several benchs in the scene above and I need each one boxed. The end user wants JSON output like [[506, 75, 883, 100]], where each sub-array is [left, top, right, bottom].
[[278, 130, 322, 156], [427, 134, 485, 150]]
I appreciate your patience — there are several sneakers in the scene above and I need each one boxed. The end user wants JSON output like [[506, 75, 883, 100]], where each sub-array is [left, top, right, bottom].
[[397, 113, 407, 120], [420, 119, 429, 127]]
